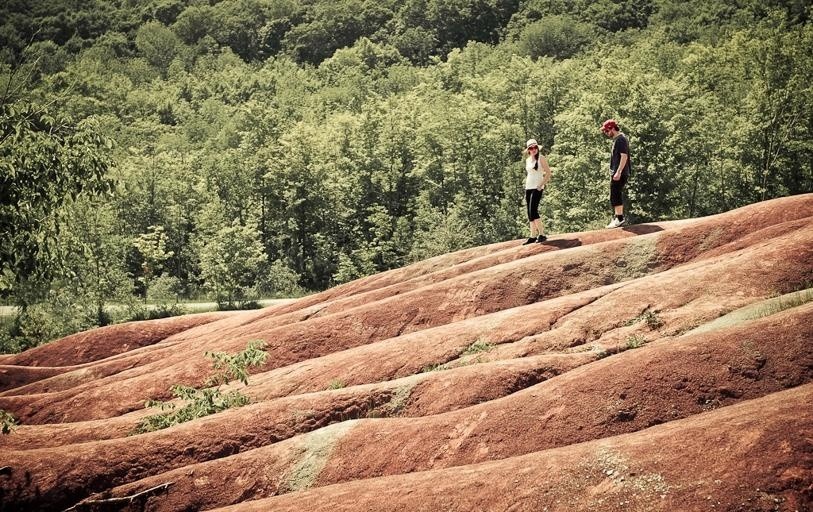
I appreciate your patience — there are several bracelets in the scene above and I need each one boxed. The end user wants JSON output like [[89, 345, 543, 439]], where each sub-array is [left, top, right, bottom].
[[616, 169, 622, 174]]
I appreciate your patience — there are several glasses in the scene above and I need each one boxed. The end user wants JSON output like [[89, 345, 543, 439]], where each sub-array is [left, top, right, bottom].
[[528, 146, 538, 150]]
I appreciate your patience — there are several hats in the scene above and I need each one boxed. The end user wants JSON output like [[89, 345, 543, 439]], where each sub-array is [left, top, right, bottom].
[[600, 119, 617, 131], [520, 139, 543, 154]]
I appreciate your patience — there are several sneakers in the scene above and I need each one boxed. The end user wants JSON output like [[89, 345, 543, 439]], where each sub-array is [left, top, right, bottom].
[[536, 235, 546, 243], [605, 216, 625, 229], [523, 237, 537, 245]]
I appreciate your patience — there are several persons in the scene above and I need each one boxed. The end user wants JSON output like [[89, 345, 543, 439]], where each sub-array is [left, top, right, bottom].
[[522, 138, 551, 245], [600, 119, 631, 228]]
